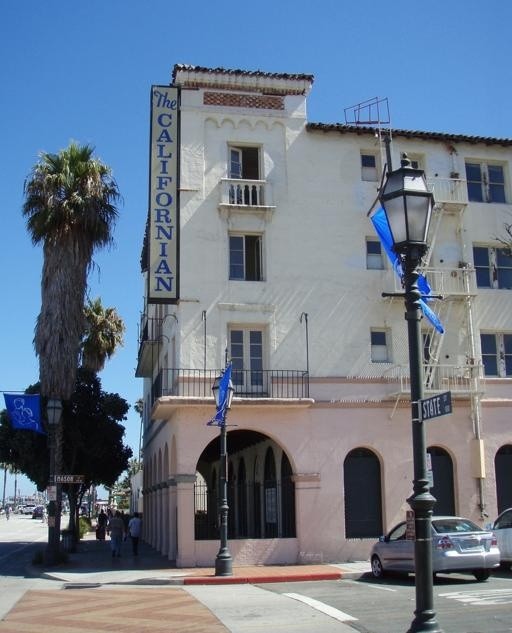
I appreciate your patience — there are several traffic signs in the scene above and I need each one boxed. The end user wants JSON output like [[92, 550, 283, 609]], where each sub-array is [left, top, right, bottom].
[[54, 474, 85, 487], [418, 391, 452, 422]]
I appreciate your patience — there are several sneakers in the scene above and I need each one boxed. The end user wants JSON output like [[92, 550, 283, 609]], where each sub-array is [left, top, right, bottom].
[[112, 550, 120, 558]]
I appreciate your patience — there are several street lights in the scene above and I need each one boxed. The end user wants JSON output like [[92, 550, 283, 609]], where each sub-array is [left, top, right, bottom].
[[380, 152, 440, 632], [212, 368, 235, 576], [47, 397, 63, 565]]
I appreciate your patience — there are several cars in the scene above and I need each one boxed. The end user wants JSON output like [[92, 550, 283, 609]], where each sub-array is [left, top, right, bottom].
[[370, 507, 511, 581], [13, 504, 47, 519]]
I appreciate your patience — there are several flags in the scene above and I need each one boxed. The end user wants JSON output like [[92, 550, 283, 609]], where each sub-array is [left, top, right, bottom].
[[204, 361, 234, 427], [2, 391, 49, 438], [367, 205, 445, 336]]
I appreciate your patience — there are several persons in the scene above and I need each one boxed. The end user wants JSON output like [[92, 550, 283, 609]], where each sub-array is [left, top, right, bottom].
[[93, 502, 144, 545], [4, 504, 12, 520], [105, 511, 127, 557], [40, 505, 48, 523], [126, 509, 144, 557]]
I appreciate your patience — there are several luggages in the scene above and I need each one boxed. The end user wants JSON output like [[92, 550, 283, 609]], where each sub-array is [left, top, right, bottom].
[[96, 528, 105, 540]]
